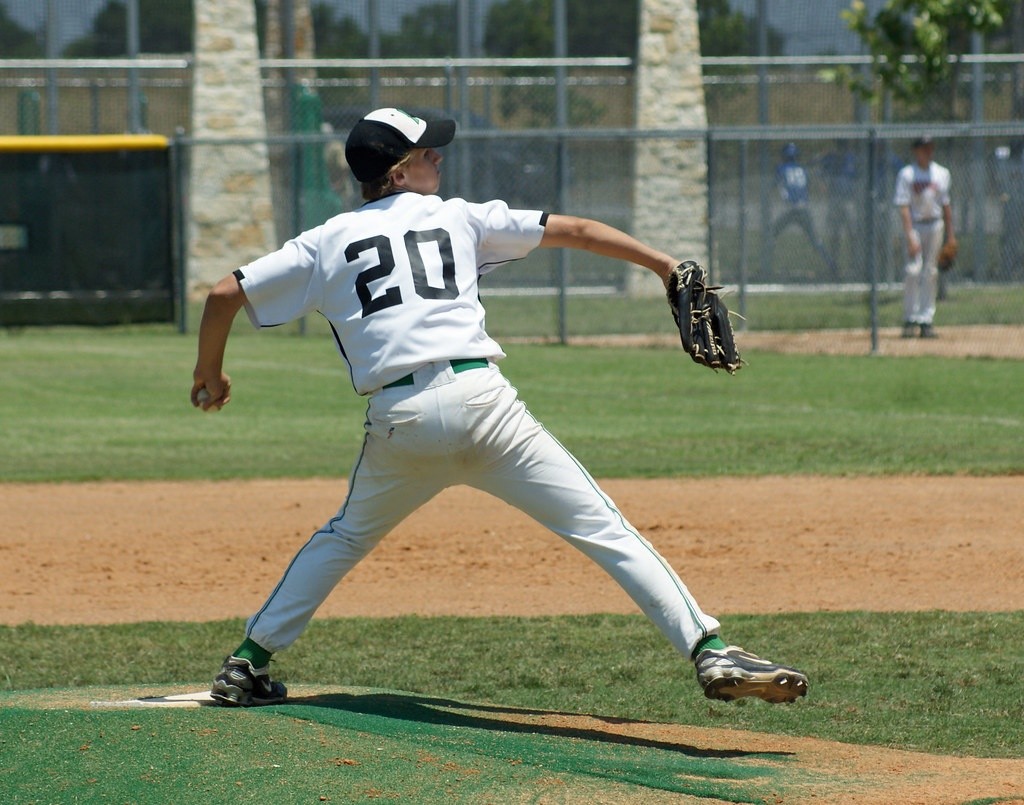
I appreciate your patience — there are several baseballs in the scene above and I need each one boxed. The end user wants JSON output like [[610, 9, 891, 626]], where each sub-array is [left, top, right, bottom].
[[197, 387, 222, 413]]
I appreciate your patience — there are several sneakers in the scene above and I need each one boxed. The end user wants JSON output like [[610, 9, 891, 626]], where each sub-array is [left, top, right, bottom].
[[694, 645, 809, 703], [210, 656, 289, 704]]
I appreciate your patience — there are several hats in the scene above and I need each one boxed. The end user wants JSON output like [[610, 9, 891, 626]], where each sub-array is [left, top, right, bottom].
[[916, 136, 933, 145], [345, 107, 456, 182], [781, 144, 801, 158]]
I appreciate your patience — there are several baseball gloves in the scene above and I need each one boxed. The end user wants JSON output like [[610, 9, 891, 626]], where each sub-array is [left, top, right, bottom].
[[939, 240, 958, 271], [665, 259, 750, 375]]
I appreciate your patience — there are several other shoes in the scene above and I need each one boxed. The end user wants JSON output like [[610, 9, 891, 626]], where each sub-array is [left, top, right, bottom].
[[921, 323, 938, 338], [903, 322, 920, 337]]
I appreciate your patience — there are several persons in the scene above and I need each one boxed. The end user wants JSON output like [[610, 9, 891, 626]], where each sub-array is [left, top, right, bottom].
[[752, 138, 1024, 282], [190, 108, 808, 707], [892, 134, 959, 339]]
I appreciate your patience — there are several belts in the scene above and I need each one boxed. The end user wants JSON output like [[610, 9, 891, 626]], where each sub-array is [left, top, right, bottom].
[[383, 355, 489, 390], [920, 218, 937, 224]]
